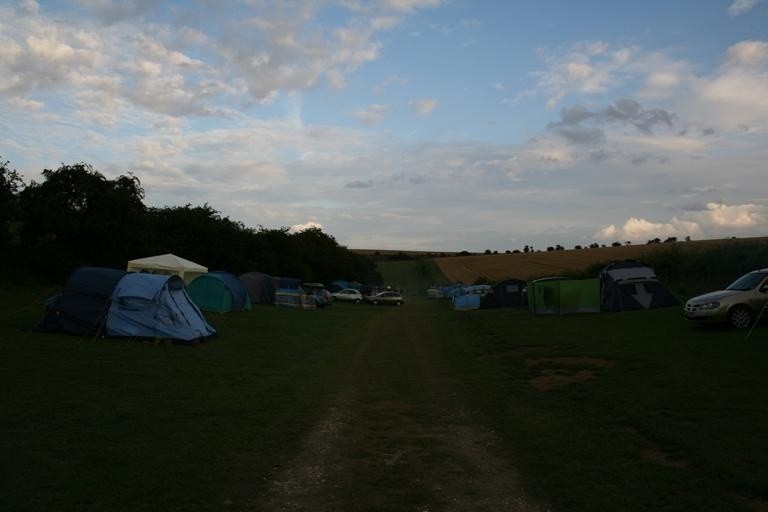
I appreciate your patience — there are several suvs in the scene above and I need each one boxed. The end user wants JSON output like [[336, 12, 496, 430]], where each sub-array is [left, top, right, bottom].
[[683, 266, 767, 331]]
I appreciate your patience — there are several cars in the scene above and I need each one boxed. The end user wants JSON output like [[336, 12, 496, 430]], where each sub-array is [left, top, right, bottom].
[[367, 291, 403, 307], [329, 287, 362, 304], [303, 283, 333, 306]]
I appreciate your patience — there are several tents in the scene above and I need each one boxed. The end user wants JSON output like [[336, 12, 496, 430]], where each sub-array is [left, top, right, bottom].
[[30, 252, 319, 343], [443, 258, 681, 317]]
